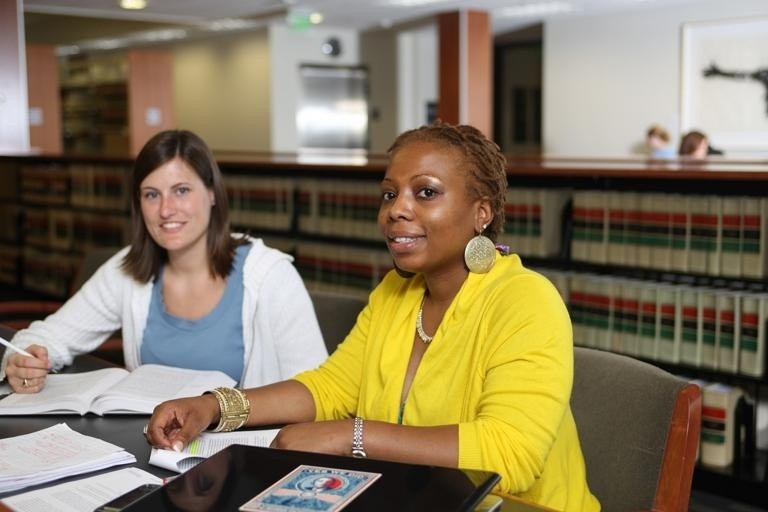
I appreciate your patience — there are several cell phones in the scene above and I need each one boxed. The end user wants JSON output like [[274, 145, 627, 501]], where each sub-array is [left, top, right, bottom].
[[102, 484, 161, 512]]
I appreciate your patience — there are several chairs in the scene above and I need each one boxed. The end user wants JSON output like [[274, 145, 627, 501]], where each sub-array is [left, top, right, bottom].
[[309, 291, 369, 355], [569, 345, 703, 512]]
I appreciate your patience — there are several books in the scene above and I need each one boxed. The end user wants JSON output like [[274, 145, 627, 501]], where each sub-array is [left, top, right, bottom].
[[498, 186, 767, 466], [0, 364, 238, 417], [0, 152, 136, 297], [223, 173, 395, 299], [237, 464, 382, 511]]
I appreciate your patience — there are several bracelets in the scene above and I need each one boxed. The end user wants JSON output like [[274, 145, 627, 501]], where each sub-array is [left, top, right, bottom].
[[204, 385, 250, 432]]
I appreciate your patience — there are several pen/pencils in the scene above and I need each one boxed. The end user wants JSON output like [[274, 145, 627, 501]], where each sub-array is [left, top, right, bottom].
[[0, 337, 58, 373]]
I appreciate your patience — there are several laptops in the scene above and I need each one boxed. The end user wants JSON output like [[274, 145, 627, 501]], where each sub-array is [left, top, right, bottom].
[[119, 444, 503, 512]]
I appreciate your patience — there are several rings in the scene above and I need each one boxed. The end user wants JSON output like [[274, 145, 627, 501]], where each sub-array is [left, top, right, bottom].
[[22, 379, 29, 389]]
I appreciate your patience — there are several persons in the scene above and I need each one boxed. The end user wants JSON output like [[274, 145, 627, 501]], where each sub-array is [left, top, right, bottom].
[[679, 130, 709, 161], [137, 123, 605, 511], [0, 127, 333, 395]]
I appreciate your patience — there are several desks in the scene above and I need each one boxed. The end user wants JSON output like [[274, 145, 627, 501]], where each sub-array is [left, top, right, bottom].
[[0, 358, 555, 512]]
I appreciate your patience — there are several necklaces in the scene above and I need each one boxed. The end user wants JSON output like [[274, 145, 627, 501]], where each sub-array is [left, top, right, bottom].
[[416, 294, 434, 342]]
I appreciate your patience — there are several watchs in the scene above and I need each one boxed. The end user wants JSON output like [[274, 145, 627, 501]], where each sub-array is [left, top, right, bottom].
[[352, 416, 367, 458]]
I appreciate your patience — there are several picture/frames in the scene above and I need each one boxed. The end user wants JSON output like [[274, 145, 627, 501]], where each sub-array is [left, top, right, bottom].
[[678, 16, 767, 153]]
